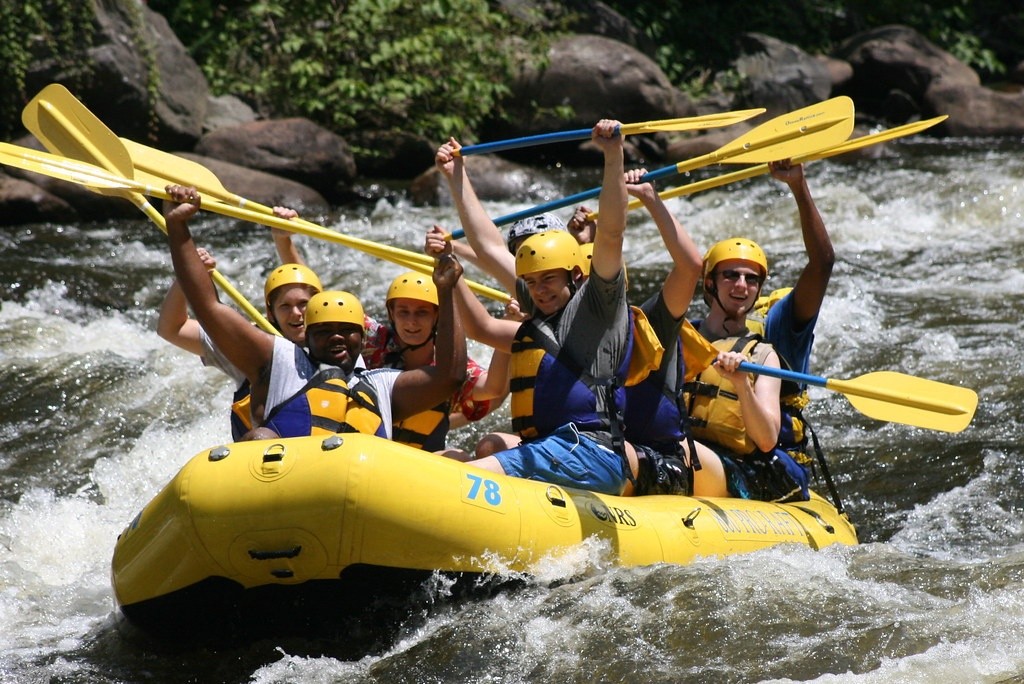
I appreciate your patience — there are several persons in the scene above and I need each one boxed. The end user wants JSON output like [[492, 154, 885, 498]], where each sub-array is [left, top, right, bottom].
[[156, 156, 834, 503], [164, 184, 463, 442], [435, 119, 634, 495]]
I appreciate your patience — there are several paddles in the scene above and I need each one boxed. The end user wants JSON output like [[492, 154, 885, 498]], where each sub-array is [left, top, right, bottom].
[[0, 140, 443, 268], [117, 136, 514, 306], [21, 83, 291, 351], [444, 107, 767, 157], [447, 96, 857, 243], [587, 115, 952, 220], [711, 350, 982, 434]]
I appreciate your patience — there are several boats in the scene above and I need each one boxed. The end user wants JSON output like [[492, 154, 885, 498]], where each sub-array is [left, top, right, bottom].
[[111, 431, 863, 651]]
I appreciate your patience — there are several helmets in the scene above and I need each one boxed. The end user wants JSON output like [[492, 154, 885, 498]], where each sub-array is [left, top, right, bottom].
[[702, 238, 767, 301], [507, 212, 570, 256], [265, 263, 323, 323], [386, 272, 439, 320], [515, 230, 585, 278], [304, 291, 365, 347], [580, 243, 628, 292]]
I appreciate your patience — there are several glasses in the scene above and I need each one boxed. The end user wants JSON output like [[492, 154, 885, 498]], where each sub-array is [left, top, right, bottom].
[[716, 270, 762, 286]]
